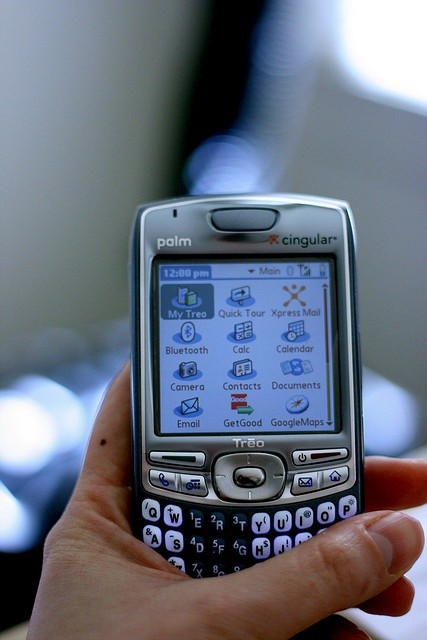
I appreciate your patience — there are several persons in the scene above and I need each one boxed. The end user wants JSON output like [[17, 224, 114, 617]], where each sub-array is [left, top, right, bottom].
[[25, 360, 425, 640]]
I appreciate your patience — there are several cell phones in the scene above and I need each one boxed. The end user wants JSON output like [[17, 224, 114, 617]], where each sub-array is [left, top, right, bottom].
[[131, 193, 365, 579]]
